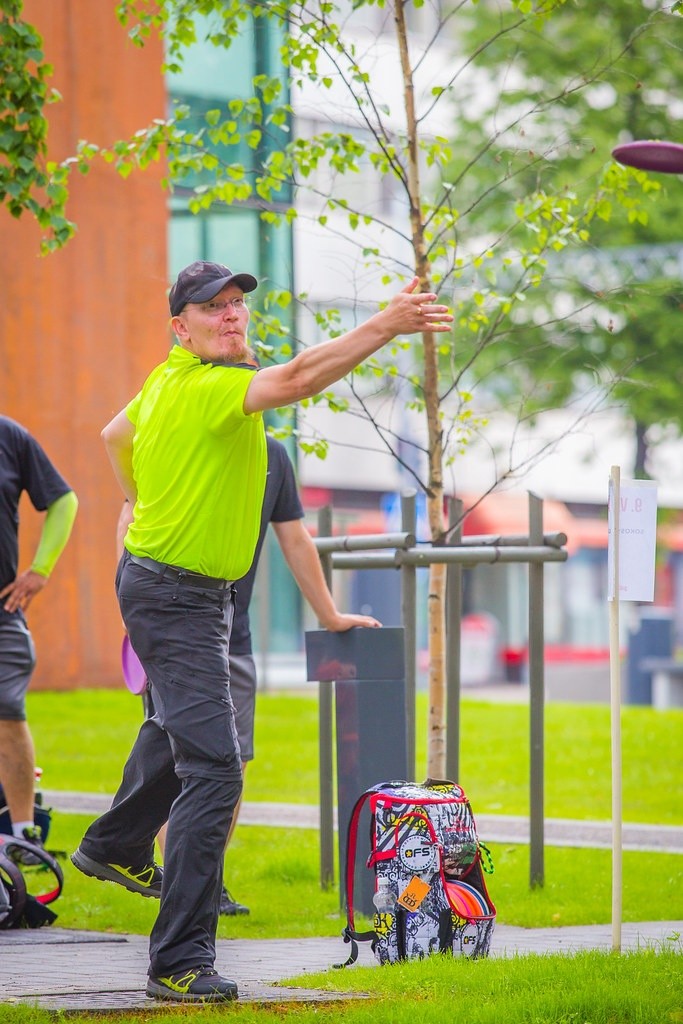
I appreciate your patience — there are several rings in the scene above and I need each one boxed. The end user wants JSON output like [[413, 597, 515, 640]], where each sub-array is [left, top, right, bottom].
[[416, 306, 422, 316]]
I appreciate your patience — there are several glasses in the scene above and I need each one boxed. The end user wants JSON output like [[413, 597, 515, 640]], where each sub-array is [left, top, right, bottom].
[[182, 296, 255, 316]]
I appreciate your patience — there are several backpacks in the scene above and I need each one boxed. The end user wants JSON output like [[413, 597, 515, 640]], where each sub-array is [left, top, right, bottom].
[[0, 783, 51, 844], [333, 779, 496, 969]]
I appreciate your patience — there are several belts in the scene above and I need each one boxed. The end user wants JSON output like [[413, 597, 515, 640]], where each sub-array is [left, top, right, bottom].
[[124, 547, 236, 591]]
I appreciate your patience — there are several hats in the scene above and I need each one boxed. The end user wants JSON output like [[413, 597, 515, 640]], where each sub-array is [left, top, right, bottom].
[[169, 260, 258, 317]]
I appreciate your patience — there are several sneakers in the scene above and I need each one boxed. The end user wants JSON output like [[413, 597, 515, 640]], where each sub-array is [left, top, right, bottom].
[[70, 847, 164, 899], [6, 825, 46, 864], [219, 886, 250, 915], [145, 966, 239, 1003]]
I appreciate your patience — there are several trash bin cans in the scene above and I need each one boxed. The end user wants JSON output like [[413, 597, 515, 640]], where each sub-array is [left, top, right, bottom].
[[627, 605, 673, 704], [651, 666, 683, 710]]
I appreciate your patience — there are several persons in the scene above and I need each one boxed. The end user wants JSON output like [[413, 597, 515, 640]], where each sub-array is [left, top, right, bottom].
[[70, 260, 454, 1004], [116, 348, 384, 915], [0, 411, 79, 866]]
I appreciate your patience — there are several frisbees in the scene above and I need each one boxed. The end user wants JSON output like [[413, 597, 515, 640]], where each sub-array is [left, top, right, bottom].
[[120, 634, 147, 697], [612, 140, 683, 173], [446, 879, 490, 918]]
